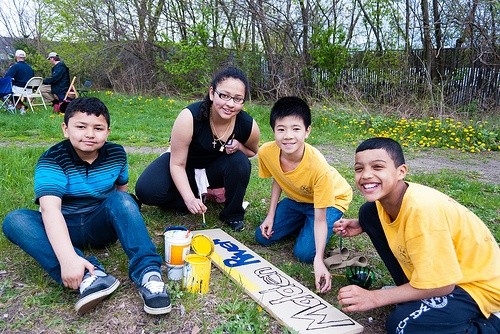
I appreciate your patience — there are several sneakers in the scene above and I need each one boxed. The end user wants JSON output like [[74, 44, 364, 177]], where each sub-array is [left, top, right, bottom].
[[229, 219, 245, 232], [74, 274, 121, 314], [139, 281, 172, 315]]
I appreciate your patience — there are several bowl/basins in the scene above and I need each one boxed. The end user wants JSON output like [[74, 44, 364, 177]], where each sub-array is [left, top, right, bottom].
[[346, 263, 374, 287]]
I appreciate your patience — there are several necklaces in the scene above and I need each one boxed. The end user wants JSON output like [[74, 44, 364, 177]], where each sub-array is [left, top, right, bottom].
[[209, 114, 233, 148], [211, 118, 236, 152]]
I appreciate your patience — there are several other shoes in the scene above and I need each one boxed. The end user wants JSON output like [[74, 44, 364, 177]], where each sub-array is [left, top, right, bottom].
[[20, 108, 26, 113], [6, 105, 14, 110]]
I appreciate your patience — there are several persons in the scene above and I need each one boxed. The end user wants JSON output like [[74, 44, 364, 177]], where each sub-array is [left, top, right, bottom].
[[4, 97, 172, 315], [257, 94, 354, 294], [135, 65, 260, 232], [2, 49, 35, 111], [332, 136, 499, 333], [40, 52, 71, 107]]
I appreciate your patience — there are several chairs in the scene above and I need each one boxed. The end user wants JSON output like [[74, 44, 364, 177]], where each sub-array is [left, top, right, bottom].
[[0, 76, 93, 115]]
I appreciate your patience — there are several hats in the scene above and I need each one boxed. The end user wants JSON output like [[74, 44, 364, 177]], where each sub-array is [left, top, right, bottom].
[[47, 52, 57, 60], [15, 50, 26, 58]]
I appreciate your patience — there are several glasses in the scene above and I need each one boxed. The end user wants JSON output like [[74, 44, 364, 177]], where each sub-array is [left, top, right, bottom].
[[214, 88, 245, 104]]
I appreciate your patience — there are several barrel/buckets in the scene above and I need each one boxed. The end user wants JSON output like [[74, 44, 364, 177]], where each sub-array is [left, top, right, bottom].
[[163, 229, 190, 263], [184, 234, 215, 293]]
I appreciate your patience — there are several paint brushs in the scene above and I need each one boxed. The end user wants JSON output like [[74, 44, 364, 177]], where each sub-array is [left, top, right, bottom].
[[339, 217, 343, 251], [350, 264, 372, 283], [201, 209, 206, 226]]
